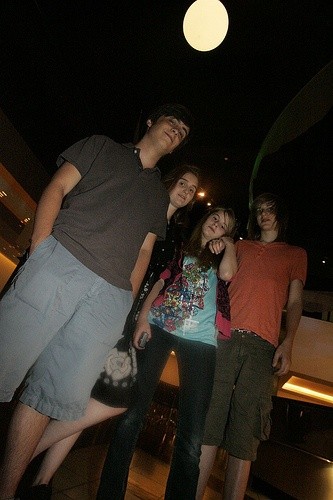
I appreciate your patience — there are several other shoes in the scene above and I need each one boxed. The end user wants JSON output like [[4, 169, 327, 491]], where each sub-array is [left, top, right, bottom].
[[29, 483, 52, 500]]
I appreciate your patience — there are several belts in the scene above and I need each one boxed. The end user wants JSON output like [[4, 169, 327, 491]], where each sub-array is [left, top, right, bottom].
[[232, 330, 255, 334]]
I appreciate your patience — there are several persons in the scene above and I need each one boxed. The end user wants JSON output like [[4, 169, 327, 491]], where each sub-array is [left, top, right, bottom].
[[93, 207, 237, 500], [0, 103, 204, 500], [195, 194, 308, 500]]
